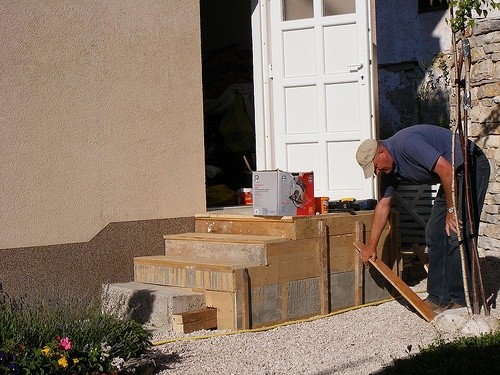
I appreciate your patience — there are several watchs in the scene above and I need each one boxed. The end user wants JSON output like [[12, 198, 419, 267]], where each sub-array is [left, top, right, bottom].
[[448, 207, 454, 214]]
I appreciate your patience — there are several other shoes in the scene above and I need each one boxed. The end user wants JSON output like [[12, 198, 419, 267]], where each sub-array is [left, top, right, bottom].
[[441, 303, 464, 312], [406, 298, 445, 311]]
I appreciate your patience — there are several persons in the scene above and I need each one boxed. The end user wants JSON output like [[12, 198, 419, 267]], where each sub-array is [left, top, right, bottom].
[[356, 125, 491, 313], [203, 77, 236, 192]]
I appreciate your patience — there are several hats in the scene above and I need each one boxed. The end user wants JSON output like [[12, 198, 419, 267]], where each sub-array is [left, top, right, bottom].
[[356, 138, 377, 179]]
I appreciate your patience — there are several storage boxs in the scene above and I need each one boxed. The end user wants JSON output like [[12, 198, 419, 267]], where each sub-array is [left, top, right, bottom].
[[252, 170, 316, 216], [237, 187, 253, 205]]
